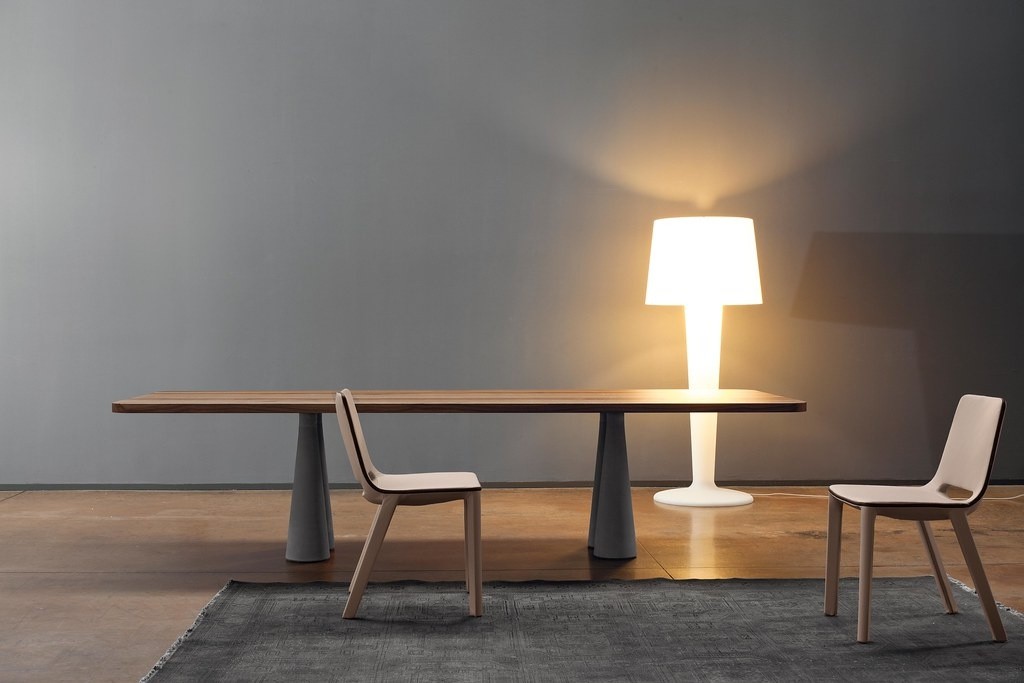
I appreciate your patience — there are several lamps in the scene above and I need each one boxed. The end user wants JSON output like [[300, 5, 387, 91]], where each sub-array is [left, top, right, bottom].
[[644, 217, 763, 506]]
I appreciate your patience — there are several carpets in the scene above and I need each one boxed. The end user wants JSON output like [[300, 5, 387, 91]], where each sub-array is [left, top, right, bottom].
[[141, 574, 1024, 683]]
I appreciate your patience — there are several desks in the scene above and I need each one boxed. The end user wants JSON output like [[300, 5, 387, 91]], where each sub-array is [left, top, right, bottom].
[[111, 389, 808, 564]]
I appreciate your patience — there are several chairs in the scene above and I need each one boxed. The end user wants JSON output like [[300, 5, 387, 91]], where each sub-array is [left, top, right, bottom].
[[824, 393, 1008, 643], [335, 389, 485, 620]]
